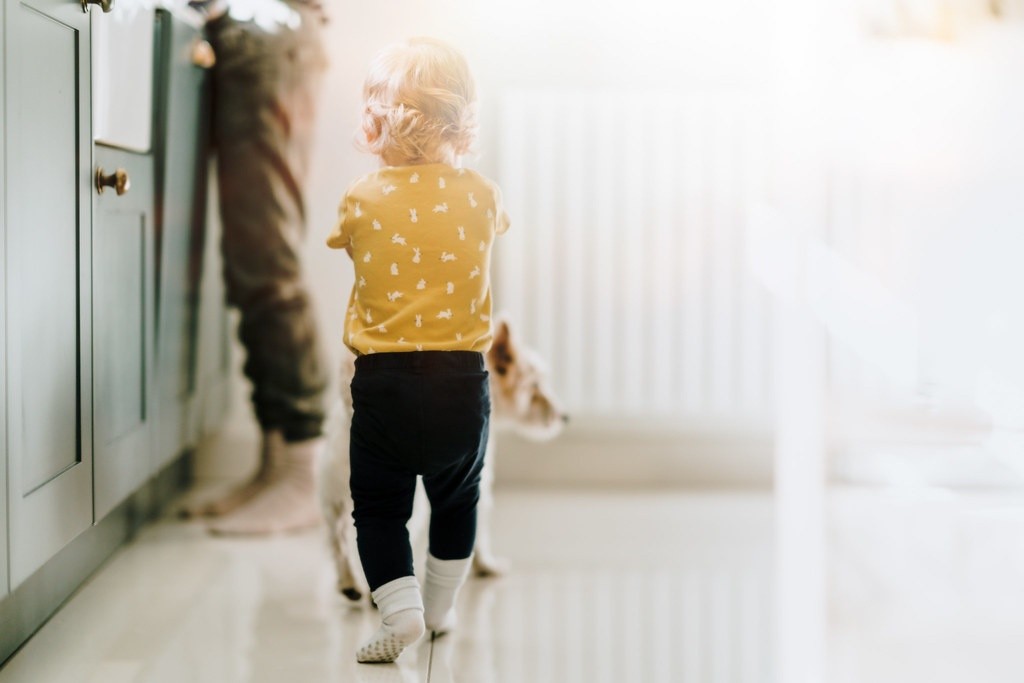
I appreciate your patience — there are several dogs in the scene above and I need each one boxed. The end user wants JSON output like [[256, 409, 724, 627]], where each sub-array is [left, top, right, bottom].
[[318, 319, 572, 610]]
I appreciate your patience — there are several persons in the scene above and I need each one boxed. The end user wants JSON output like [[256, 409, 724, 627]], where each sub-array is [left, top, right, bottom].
[[171, 0, 350, 535], [327, 34, 513, 664]]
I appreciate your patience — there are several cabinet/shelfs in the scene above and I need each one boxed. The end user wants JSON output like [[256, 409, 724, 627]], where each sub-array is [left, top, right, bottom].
[[0, 1, 244, 664]]
